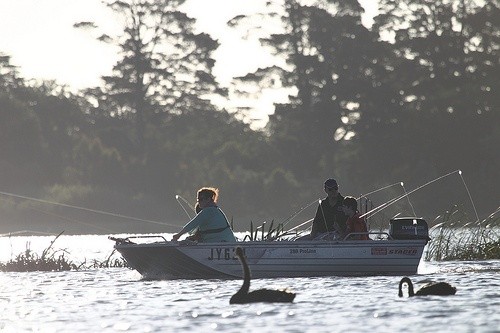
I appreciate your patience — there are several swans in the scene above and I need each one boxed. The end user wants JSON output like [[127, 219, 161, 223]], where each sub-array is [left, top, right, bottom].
[[228, 246, 297, 304], [398, 276, 458, 298]]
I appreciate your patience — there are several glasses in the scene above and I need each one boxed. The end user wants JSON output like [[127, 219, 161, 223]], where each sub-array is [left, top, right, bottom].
[[197, 198, 207, 202]]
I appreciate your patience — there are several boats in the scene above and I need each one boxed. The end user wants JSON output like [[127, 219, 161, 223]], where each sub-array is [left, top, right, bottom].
[[109, 218, 429, 279]]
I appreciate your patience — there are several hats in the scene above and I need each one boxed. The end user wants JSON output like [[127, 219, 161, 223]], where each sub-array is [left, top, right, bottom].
[[324, 178, 337, 188]]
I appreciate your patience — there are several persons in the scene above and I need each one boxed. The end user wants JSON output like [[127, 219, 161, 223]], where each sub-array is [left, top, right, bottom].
[[172, 187, 237, 242], [183, 203, 201, 242], [332, 195, 369, 240], [294, 178, 349, 241]]
